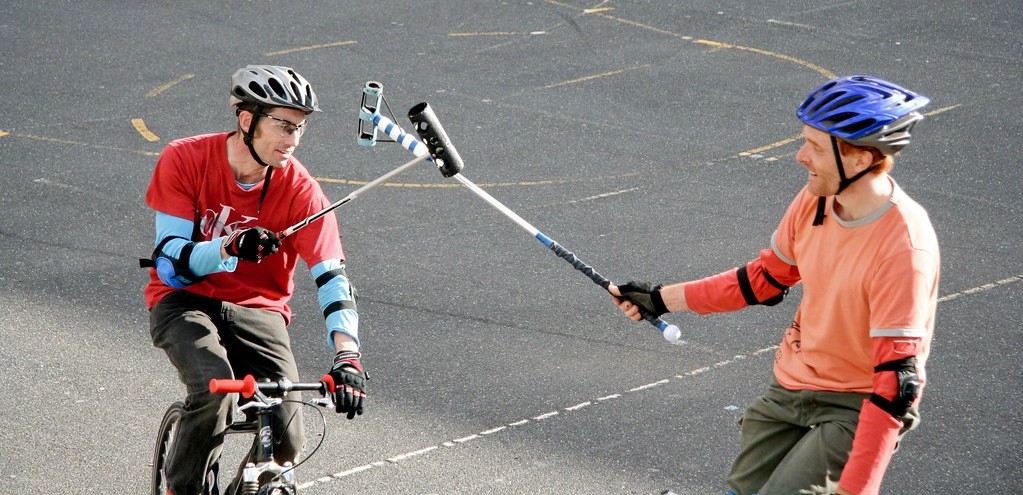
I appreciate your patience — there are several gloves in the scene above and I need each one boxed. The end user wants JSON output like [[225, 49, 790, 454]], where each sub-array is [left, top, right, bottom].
[[224, 225, 283, 264], [317, 349, 370, 418]]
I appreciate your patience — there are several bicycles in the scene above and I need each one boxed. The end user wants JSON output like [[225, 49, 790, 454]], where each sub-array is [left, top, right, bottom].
[[149, 369, 372, 495]]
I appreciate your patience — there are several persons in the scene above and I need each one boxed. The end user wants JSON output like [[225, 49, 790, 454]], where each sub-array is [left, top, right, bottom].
[[608, 73, 940, 495], [141, 65, 364, 495]]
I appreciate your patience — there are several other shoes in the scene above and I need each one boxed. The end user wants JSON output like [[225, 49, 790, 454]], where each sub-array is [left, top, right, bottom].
[[276, 455, 301, 470]]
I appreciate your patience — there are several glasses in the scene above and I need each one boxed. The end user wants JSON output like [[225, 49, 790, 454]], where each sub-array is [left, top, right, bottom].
[[260, 114, 310, 137]]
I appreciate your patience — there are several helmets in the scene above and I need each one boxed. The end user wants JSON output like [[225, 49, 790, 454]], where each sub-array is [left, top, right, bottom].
[[796, 74, 929, 157], [229, 64, 322, 115]]
